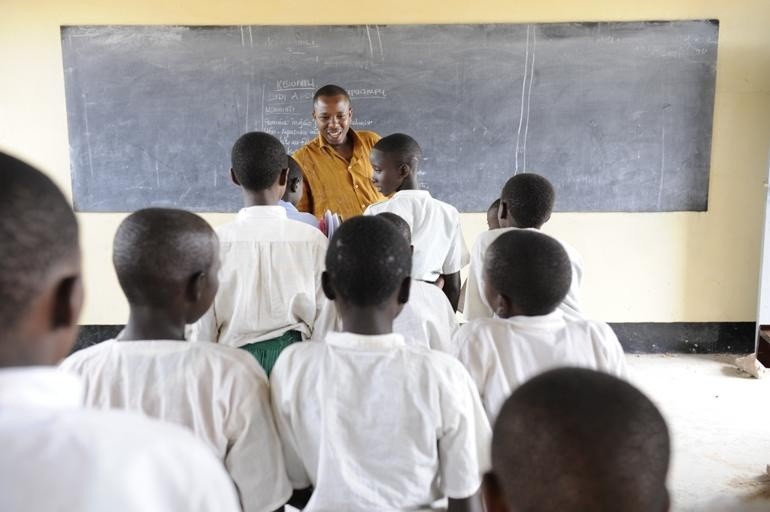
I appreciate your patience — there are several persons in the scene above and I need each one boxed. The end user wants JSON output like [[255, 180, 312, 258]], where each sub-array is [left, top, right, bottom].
[[362, 132, 471, 314], [186, 130, 331, 375], [0, 149, 241, 512], [291, 83, 398, 228], [450, 229, 632, 433], [480, 364, 673, 512], [309, 212, 458, 352], [456, 196, 501, 315], [268, 214, 495, 512], [462, 173, 589, 320], [276, 154, 324, 227], [58, 206, 295, 512]]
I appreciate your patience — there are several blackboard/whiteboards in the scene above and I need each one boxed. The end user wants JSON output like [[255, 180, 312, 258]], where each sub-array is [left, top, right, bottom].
[[60, 18, 719, 212]]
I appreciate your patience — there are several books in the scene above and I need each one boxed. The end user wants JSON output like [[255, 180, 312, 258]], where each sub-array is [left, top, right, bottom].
[[319, 209, 344, 240]]
